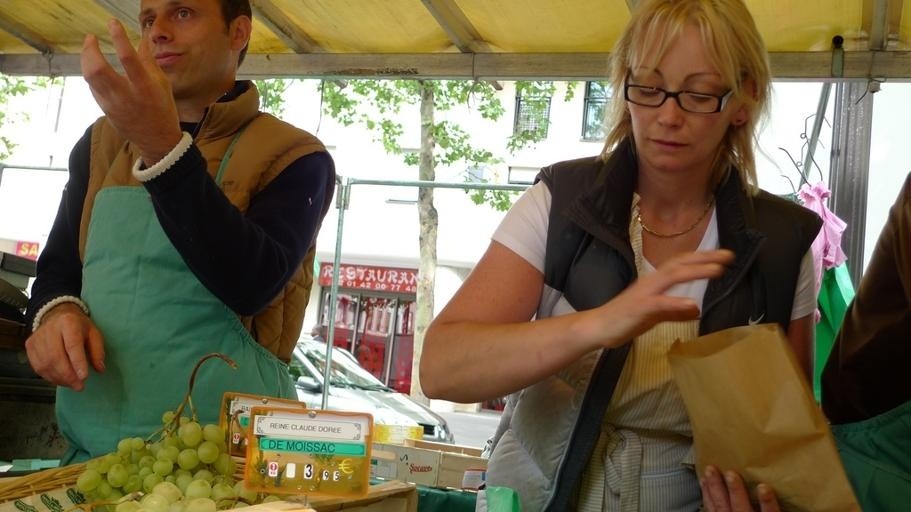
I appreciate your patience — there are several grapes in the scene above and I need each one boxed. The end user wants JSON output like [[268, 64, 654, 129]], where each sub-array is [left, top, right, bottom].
[[73, 411, 280, 512]]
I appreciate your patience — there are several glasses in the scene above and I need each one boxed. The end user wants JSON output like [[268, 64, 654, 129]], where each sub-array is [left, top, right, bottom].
[[621, 65, 742, 115]]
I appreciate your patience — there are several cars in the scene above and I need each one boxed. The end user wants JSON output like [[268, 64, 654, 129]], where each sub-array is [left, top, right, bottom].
[[288, 338, 454, 445]]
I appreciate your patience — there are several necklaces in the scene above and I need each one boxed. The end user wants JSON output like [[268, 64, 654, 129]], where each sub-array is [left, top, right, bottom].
[[638, 190, 716, 241]]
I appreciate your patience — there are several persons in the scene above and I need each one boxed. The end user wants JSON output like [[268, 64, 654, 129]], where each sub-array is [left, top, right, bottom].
[[822, 172, 911, 512], [25, 0, 337, 465], [418, 0, 823, 512]]
